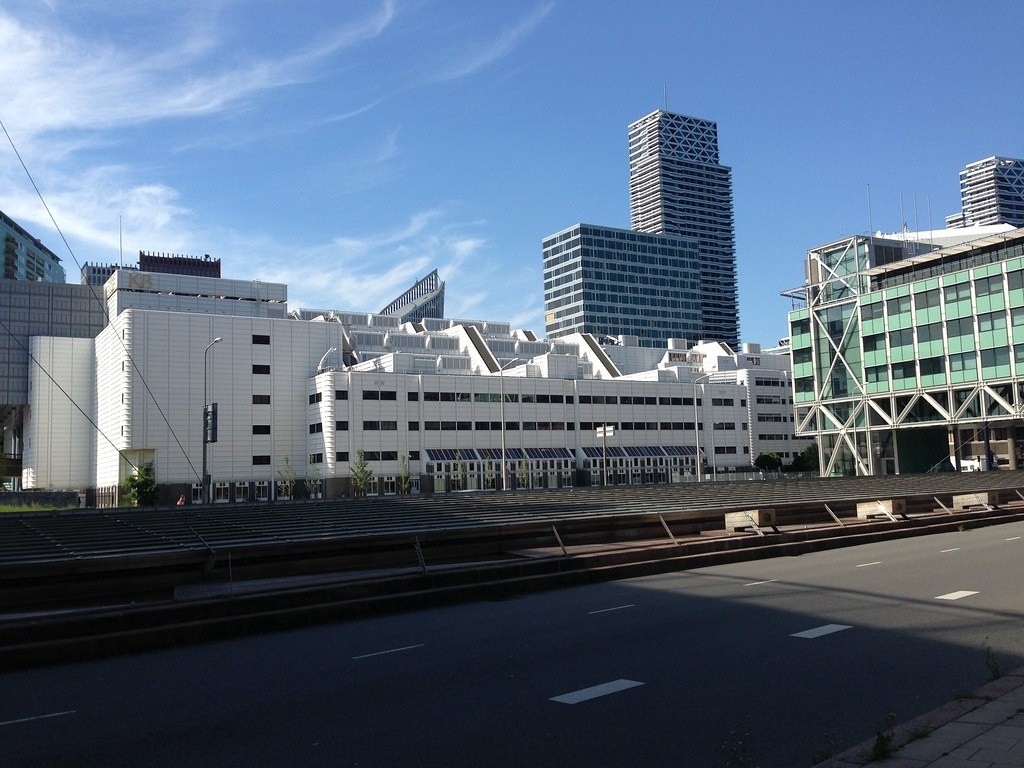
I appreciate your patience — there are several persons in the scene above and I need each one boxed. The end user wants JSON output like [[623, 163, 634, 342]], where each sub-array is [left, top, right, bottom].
[[77, 496, 82, 507]]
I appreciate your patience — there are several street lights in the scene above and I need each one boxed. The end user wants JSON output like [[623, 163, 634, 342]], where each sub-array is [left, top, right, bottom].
[[492, 357, 529, 491], [681, 372, 716, 482], [852, 380, 869, 478], [202, 337, 230, 506]]
